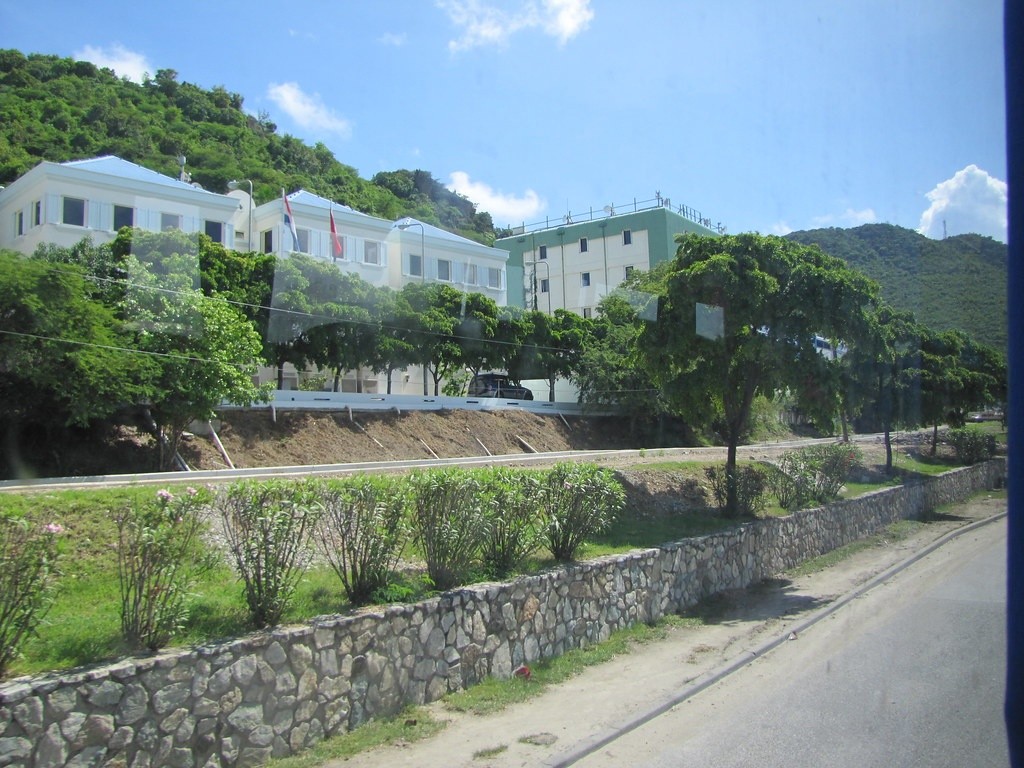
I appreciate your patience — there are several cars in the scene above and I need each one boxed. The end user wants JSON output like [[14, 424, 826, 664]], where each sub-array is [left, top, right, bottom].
[[961, 408, 1004, 423]]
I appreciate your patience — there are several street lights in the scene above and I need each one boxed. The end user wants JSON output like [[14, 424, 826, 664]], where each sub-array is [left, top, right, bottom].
[[397, 223, 425, 284], [525, 261, 551, 316], [226, 178, 252, 254]]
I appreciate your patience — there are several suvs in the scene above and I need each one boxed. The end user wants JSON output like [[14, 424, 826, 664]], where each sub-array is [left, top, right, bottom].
[[467, 373, 534, 401]]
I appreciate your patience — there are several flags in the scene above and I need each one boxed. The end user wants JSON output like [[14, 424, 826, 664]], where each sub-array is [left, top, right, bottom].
[[330, 210, 341, 258], [283, 194, 300, 254]]
